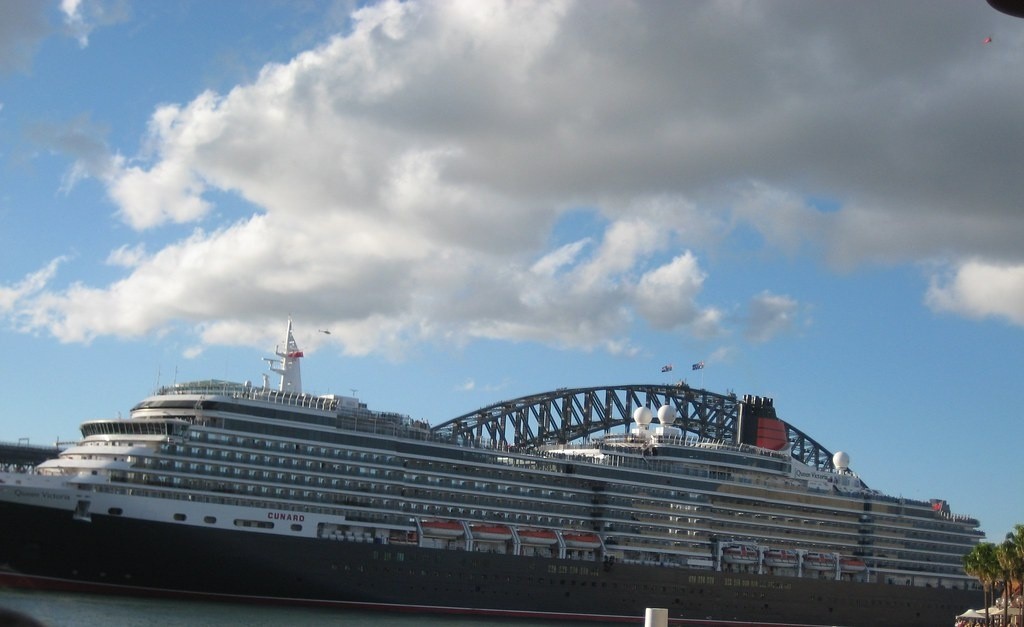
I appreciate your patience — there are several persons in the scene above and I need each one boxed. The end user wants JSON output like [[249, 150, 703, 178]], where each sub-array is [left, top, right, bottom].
[[954, 617, 1020, 627]]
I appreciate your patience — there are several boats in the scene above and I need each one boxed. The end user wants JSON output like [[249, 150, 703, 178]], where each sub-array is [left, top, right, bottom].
[[562, 535, 601, 548], [517, 531, 558, 545], [724, 546, 758, 564], [421, 522, 464, 536], [840, 560, 864, 571], [764, 549, 799, 567], [802, 552, 835, 571], [472, 526, 512, 540]]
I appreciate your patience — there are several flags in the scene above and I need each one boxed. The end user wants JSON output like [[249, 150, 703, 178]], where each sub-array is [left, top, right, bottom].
[[662, 365, 672, 372], [693, 361, 704, 370]]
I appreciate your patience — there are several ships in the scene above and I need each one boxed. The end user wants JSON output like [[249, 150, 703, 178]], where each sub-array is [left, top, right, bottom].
[[0, 313, 1004, 627]]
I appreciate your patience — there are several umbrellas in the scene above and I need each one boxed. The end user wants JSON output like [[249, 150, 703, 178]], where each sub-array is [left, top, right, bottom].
[[956, 607, 1022, 619]]
